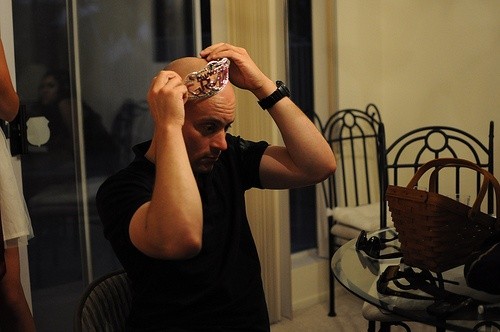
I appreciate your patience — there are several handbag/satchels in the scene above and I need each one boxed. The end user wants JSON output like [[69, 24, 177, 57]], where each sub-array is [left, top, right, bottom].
[[386, 158, 500, 269]]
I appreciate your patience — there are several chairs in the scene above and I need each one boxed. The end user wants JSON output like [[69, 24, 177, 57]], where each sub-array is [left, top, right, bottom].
[[77, 270, 134, 332], [311, 103, 494, 332]]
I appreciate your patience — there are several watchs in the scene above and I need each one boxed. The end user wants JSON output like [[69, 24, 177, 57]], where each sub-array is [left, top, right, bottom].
[[256, 80, 292, 111]]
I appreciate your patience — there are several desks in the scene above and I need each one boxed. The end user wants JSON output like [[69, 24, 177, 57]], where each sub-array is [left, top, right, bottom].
[[331, 226, 500, 332]]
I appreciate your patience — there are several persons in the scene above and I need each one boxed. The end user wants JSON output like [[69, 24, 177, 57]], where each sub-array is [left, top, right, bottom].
[[96, 41, 338, 332], [0, 36, 35, 332]]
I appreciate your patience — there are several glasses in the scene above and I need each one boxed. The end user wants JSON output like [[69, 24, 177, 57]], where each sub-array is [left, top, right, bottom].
[[355, 229, 402, 259], [377, 265, 460, 300]]
